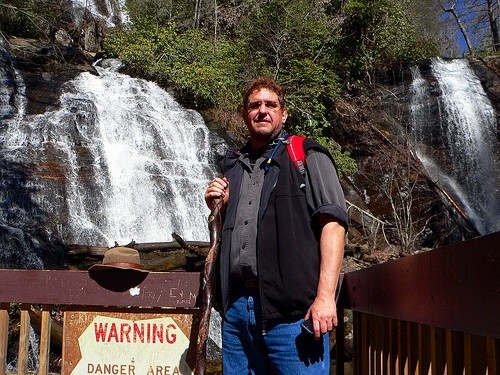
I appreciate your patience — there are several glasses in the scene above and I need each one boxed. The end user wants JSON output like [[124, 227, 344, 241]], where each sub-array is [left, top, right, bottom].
[[246, 101, 282, 109]]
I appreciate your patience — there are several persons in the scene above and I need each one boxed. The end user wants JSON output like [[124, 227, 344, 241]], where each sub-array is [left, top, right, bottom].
[[203, 76, 351, 375]]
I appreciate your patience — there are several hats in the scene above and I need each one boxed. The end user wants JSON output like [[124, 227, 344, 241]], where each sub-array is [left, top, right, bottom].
[[87, 246, 150, 274]]
